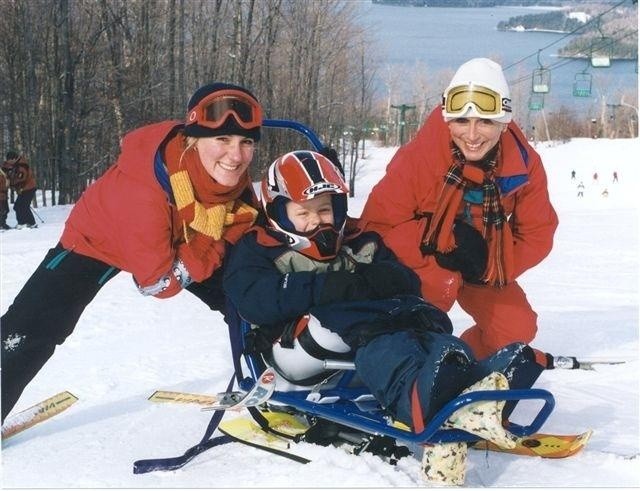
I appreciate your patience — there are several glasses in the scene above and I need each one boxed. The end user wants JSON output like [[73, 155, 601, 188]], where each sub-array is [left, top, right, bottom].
[[447, 86, 511, 115], [186, 90, 263, 130]]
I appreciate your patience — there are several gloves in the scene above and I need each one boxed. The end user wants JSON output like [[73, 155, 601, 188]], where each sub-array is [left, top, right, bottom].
[[432, 222, 489, 283]]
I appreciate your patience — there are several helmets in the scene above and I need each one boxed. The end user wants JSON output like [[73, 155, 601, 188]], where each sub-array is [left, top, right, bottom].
[[261, 149, 348, 261]]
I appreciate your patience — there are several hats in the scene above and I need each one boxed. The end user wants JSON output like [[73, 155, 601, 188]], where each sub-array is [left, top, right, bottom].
[[183, 83, 263, 140], [441, 58, 514, 123]]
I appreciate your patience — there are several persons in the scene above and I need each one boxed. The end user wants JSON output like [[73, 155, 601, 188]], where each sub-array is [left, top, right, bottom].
[[571, 170, 619, 197], [225, 149, 538, 428], [355, 56, 560, 362], [1, 81, 263, 427], [0, 160, 16, 229], [6, 151, 38, 229]]
